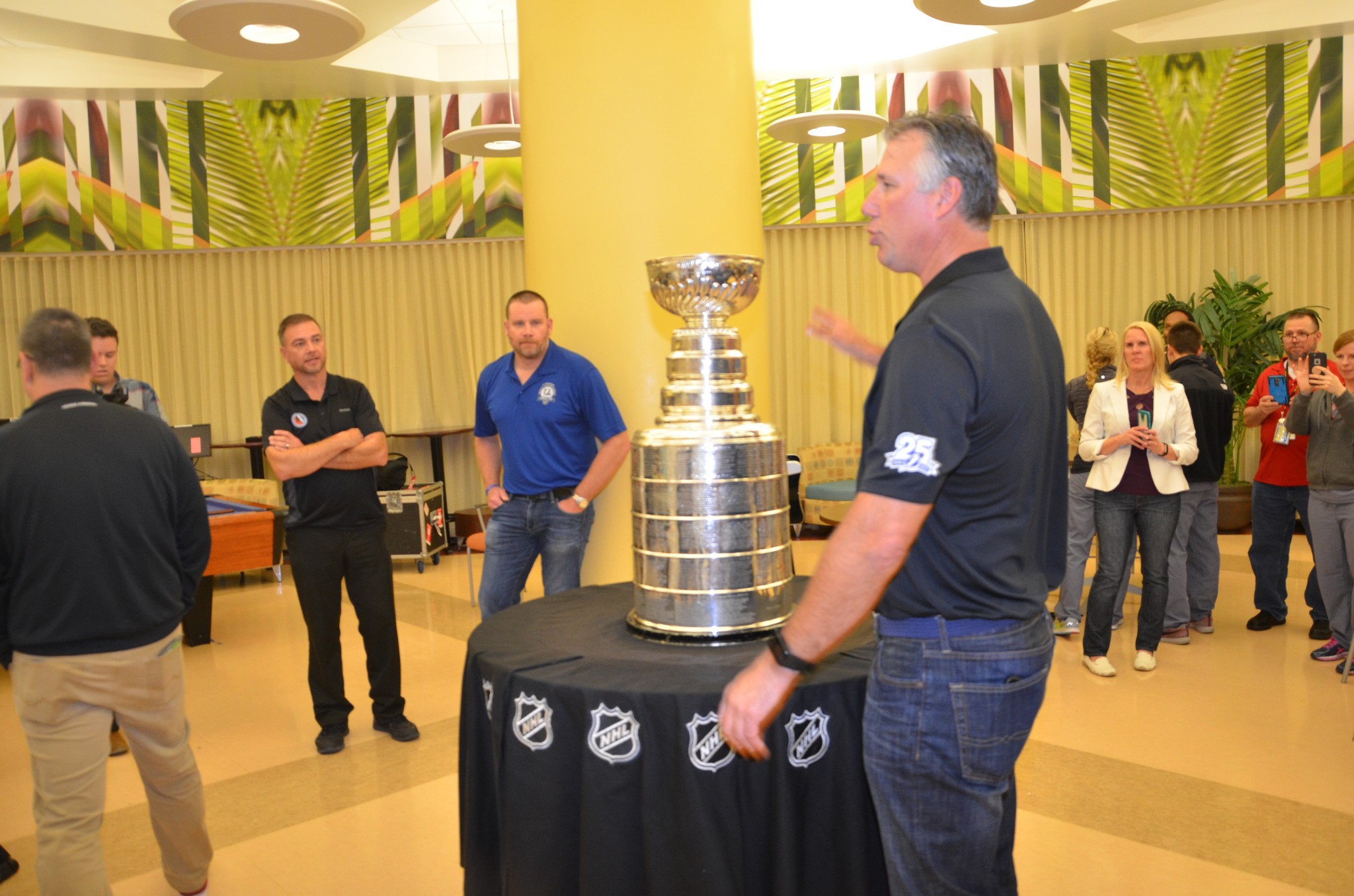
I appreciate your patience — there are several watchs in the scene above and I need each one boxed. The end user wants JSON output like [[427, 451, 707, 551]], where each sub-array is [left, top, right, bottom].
[[1159, 443, 1168, 456], [571, 491, 589, 510], [766, 630, 819, 673]]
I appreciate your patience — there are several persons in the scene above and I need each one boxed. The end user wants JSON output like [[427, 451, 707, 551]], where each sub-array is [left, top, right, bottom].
[[0, 308, 212, 896], [718, 111, 1070, 896], [1063, 306, 1354, 677], [471, 291, 632, 626], [260, 315, 419, 755]]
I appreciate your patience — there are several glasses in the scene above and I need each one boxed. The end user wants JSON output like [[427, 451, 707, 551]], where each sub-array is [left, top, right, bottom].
[[1282, 330, 1318, 343]]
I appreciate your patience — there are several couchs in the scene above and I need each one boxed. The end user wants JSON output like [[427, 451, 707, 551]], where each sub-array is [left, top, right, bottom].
[[794, 439, 862, 532], [199, 476, 289, 512]]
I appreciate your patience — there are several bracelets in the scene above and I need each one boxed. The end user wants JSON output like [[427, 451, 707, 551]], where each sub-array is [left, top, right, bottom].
[[485, 484, 500, 495]]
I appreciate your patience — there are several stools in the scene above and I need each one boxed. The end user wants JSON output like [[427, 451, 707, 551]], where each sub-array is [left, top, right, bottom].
[[452, 506, 493, 552]]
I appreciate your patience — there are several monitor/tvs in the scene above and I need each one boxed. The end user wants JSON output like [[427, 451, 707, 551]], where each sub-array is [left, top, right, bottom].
[[169, 424, 212, 458]]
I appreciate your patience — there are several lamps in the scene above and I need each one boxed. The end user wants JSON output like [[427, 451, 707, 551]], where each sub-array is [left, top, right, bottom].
[[764, 77, 891, 146], [164, 0, 368, 65], [911, 0, 1094, 26], [440, 9, 522, 159]]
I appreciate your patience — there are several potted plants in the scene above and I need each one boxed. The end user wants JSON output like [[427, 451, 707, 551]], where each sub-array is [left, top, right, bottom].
[[1139, 266, 1327, 536]]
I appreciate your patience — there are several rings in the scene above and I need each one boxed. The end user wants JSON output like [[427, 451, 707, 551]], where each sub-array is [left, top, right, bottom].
[[287, 443, 290, 448]]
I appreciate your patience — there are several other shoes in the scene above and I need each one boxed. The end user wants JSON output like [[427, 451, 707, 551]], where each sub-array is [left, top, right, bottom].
[[1052, 617, 1081, 635], [1207, 622, 1213, 624], [109, 731, 127, 755], [1082, 653, 1117, 676], [1309, 619, 1333, 639], [1134, 651, 1158, 671], [1111, 618, 1122, 630], [0, 858, 16, 883], [1246, 608, 1286, 632], [1160, 627, 1191, 644]]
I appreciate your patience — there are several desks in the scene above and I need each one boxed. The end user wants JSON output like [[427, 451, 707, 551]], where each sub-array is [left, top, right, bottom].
[[206, 441, 269, 482], [392, 422, 477, 549], [452, 578, 893, 896], [181, 491, 291, 649]]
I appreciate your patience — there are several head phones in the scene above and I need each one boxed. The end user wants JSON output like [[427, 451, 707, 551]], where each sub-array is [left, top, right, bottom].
[[95, 382, 128, 404]]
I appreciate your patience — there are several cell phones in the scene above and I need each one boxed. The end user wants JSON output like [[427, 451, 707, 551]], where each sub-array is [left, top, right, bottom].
[[1268, 376, 1289, 405], [1138, 410, 1151, 441], [1309, 352, 1327, 385]]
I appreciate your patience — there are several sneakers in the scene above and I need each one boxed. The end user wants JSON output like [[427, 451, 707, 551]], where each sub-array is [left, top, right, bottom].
[[1336, 658, 1354, 675], [314, 723, 349, 754], [1188, 617, 1215, 633], [374, 714, 419, 742], [1311, 636, 1351, 661]]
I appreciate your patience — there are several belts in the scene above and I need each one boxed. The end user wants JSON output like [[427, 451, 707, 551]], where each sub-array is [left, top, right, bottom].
[[518, 487, 574, 501]]
